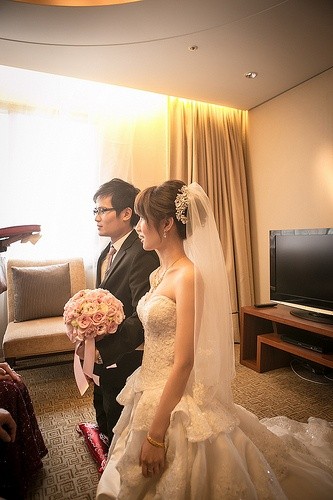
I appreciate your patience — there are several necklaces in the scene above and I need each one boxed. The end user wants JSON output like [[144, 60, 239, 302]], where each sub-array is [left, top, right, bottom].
[[151, 255, 186, 293]]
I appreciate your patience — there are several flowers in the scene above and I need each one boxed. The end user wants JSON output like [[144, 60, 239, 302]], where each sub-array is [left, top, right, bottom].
[[63, 288, 126, 335]]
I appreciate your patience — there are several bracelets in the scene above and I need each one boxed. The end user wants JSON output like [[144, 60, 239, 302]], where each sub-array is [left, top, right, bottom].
[[96, 348, 103, 364], [146, 432, 164, 448]]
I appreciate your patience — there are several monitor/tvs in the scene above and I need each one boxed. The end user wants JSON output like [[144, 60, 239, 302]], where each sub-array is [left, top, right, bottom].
[[269, 228, 333, 324]]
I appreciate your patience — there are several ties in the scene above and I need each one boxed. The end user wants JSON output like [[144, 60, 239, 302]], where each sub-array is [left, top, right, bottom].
[[103, 246, 117, 282]]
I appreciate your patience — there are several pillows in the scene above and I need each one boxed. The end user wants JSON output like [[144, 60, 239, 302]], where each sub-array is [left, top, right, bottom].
[[11, 263, 71, 323]]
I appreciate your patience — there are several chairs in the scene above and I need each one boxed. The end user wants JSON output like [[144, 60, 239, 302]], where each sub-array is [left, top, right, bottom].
[[2, 258, 84, 372]]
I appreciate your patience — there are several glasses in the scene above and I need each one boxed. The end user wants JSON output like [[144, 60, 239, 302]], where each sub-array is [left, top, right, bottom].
[[93, 207, 115, 215]]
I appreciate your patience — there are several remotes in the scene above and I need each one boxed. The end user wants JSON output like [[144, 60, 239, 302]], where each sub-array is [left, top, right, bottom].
[[254, 303, 278, 308]]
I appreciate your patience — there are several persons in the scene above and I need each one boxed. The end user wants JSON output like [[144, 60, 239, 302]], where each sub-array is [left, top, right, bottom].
[[0, 232, 48, 500], [96, 180, 333, 500], [76, 177, 160, 456]]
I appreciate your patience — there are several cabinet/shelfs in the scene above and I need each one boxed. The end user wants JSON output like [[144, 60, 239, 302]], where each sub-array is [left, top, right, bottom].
[[239, 303, 333, 374]]
